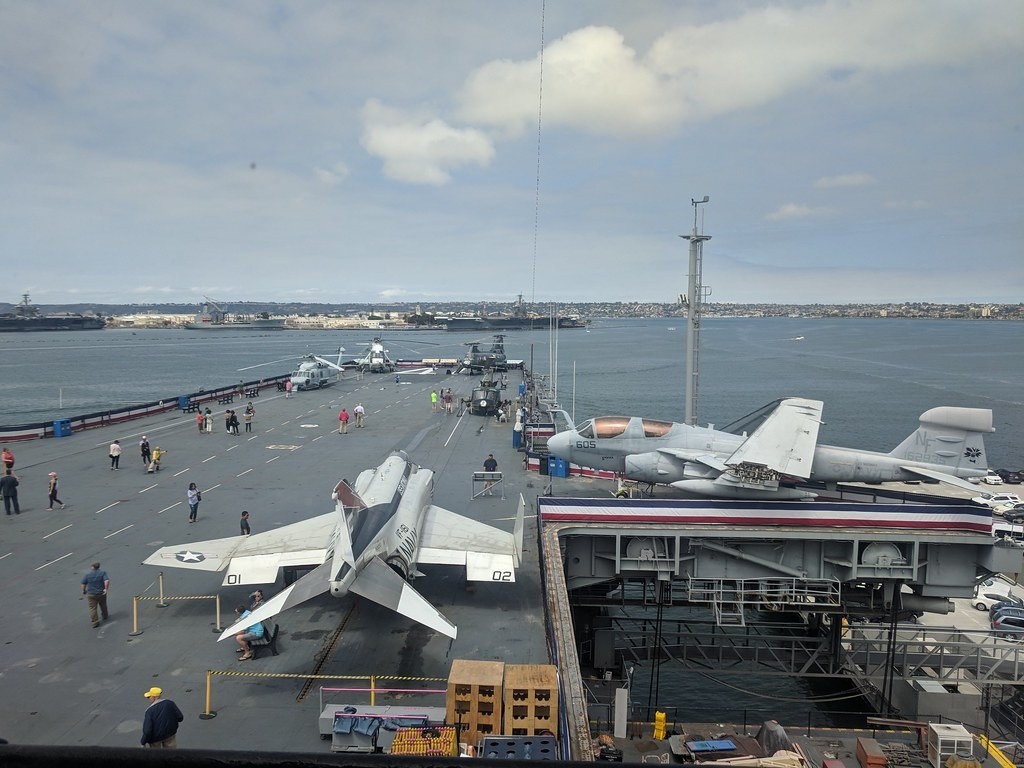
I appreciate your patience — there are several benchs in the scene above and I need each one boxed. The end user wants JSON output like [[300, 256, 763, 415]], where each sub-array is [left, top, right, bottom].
[[245, 388, 259, 398], [248, 618, 279, 661], [217, 393, 234, 405], [182, 401, 200, 413]]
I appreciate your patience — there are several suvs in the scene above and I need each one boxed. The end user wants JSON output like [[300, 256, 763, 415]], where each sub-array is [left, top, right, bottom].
[[972, 575, 1024, 644]]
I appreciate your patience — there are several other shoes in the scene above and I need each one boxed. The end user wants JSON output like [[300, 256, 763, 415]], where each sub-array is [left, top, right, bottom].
[[188, 519, 193, 523], [193, 518, 199, 522], [46, 508, 53, 510], [61, 504, 65, 509]]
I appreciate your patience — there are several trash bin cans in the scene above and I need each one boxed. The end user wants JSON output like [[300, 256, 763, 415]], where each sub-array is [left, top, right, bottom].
[[540, 454, 548, 475], [178, 394, 191, 410], [513, 428, 522, 449], [53, 417, 72, 437], [548, 453, 557, 477], [557, 453, 569, 478], [332, 712, 428, 754], [517, 383, 526, 399]]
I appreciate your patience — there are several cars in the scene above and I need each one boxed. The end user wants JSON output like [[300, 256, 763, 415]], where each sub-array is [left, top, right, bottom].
[[959, 466, 1024, 524]]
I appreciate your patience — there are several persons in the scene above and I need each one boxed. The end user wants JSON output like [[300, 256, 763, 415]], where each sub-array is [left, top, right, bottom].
[[225, 409, 240, 436], [439, 387, 454, 414], [239, 380, 250, 399], [2, 448, 20, 479], [109, 440, 122, 471], [286, 379, 293, 398], [483, 453, 498, 497], [139, 435, 151, 467], [137, 686, 185, 748], [244, 402, 255, 433], [240, 511, 251, 535], [187, 483, 201, 524], [338, 407, 349, 435], [152, 446, 165, 470], [431, 390, 437, 414], [231, 606, 265, 660], [0, 469, 20, 515], [380, 362, 384, 373], [44, 472, 66, 511], [493, 398, 511, 423], [432, 363, 439, 376], [80, 562, 109, 628], [842, 616, 849, 637], [354, 402, 365, 429], [249, 590, 264, 612], [394, 374, 401, 386], [197, 407, 214, 434], [355, 363, 361, 382]]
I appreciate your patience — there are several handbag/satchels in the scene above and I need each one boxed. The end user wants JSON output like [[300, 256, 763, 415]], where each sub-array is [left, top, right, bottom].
[[195, 488, 201, 501]]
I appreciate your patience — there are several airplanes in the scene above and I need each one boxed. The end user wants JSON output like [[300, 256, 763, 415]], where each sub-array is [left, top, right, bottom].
[[546, 395, 995, 499], [461, 365, 507, 416], [141, 449, 526, 640]]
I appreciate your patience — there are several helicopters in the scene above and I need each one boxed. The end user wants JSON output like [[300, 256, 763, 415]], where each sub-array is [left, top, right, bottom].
[[348, 331, 440, 373], [415, 334, 547, 376], [236, 343, 366, 392]]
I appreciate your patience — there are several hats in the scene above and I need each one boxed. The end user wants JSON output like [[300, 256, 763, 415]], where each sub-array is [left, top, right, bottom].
[[144, 687, 163, 697], [48, 472, 57, 477]]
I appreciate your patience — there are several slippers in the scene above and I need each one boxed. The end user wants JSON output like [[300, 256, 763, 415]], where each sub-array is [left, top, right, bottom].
[[238, 654, 252, 661], [236, 648, 245, 652]]
